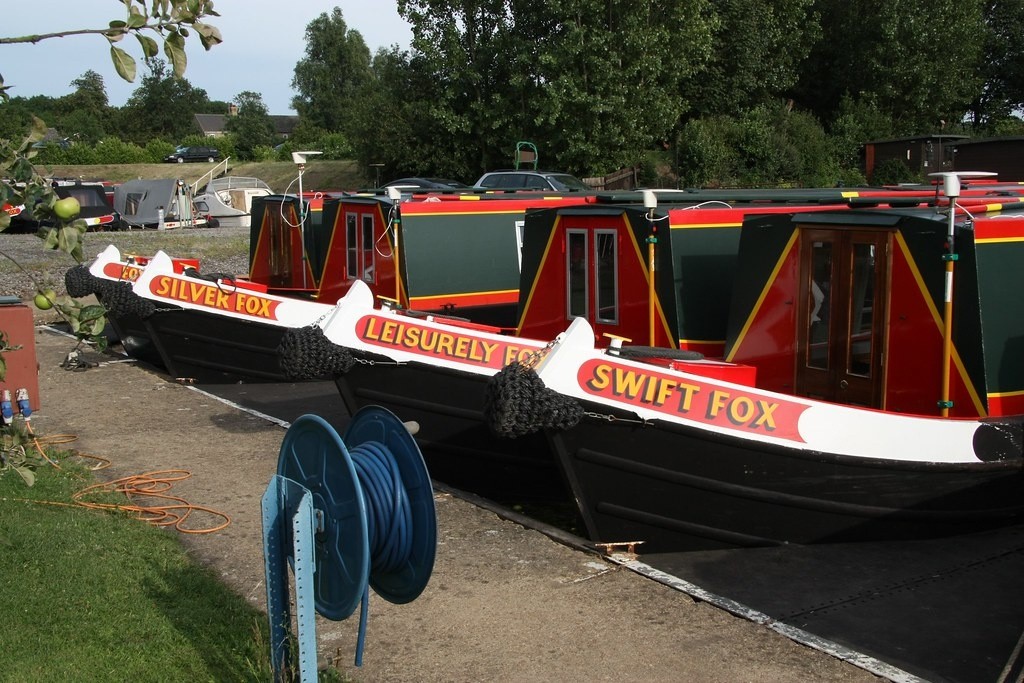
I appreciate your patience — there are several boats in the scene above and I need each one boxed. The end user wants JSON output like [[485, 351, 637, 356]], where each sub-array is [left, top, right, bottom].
[[0, 119, 1024, 554]]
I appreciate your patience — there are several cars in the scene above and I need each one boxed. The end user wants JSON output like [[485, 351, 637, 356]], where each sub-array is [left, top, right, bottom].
[[376, 174, 469, 189]]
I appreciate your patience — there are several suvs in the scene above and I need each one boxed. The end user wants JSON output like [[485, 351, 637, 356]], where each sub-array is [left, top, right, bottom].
[[471, 167, 595, 191]]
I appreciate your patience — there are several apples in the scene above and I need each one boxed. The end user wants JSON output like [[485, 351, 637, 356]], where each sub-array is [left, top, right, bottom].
[[35, 288, 55, 309], [54, 197, 80, 222]]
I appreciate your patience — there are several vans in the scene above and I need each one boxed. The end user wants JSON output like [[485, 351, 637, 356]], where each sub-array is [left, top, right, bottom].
[[163, 147, 220, 163]]
